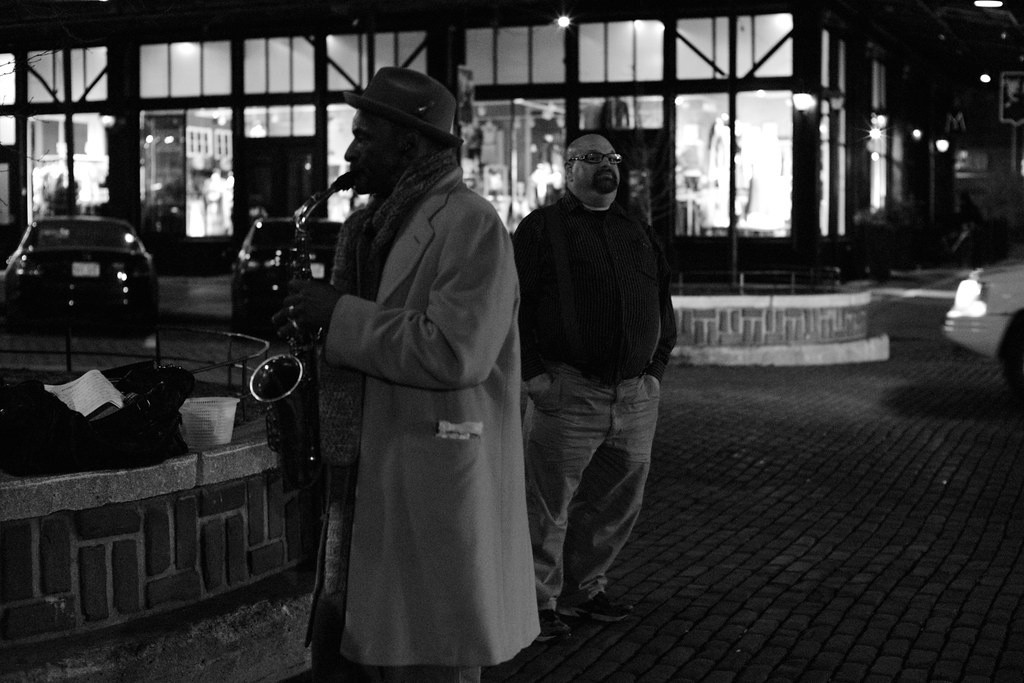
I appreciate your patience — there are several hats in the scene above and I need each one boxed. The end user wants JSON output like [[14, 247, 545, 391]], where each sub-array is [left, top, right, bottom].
[[344, 67, 465, 146]]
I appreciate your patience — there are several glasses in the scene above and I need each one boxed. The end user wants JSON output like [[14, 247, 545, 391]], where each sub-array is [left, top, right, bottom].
[[569, 152, 622, 164]]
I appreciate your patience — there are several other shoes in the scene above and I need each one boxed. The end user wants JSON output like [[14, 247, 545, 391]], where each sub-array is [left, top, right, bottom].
[[561, 592, 629, 621], [534, 609, 571, 643]]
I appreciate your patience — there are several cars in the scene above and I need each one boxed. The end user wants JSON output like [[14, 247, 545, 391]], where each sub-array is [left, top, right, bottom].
[[0, 214, 158, 332], [940, 248, 1024, 395]]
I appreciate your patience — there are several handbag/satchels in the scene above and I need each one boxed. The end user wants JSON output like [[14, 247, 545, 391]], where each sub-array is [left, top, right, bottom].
[[0, 360, 193, 478]]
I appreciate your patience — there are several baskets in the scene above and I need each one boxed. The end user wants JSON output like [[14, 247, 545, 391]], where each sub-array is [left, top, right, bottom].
[[179, 397, 240, 449]]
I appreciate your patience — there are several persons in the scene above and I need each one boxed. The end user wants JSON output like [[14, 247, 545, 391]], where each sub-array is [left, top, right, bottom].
[[513, 133, 679, 644], [281, 66, 543, 683]]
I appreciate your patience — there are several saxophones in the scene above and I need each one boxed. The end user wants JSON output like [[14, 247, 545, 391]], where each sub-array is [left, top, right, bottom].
[[248, 170, 357, 495]]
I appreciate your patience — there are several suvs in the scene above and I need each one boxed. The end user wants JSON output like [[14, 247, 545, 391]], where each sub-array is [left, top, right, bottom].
[[231, 217, 346, 335]]
